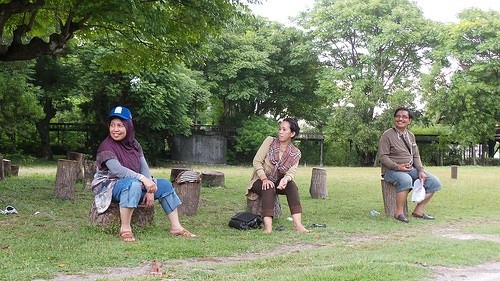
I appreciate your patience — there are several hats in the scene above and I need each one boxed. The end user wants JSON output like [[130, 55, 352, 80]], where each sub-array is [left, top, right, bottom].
[[107, 106, 132, 121], [412, 179, 426, 203]]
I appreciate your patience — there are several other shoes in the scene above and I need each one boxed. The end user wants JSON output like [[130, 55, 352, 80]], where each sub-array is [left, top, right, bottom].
[[274, 225, 285, 231], [411, 210, 435, 220], [394, 213, 409, 223], [310, 223, 326, 228]]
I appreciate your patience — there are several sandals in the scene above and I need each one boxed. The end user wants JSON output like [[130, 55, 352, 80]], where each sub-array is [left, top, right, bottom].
[[168, 226, 197, 238], [118, 230, 136, 242]]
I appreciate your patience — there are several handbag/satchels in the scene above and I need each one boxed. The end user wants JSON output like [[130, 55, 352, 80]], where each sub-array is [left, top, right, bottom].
[[228, 211, 262, 231]]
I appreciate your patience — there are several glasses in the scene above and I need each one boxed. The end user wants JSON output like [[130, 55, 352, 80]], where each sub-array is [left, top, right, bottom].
[[396, 114, 410, 119]]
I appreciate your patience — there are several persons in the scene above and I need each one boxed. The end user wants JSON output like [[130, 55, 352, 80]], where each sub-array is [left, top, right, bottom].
[[244, 118, 314, 234], [92, 106, 196, 242], [377, 108, 441, 222]]
